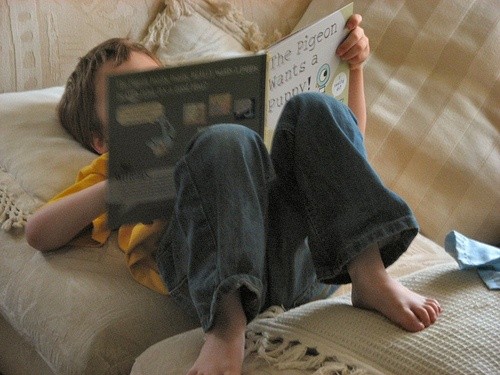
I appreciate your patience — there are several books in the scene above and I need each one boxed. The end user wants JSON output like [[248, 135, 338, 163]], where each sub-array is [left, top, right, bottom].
[[106, 1, 358, 227]]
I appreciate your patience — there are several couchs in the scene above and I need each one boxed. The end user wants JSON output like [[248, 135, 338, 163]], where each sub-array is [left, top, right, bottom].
[[0, 0, 500, 375]]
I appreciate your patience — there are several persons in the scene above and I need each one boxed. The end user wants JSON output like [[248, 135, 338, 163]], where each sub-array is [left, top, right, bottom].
[[27, 14, 441, 375]]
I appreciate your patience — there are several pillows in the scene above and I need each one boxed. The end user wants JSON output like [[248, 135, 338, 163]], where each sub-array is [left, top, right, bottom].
[[139, 0, 269, 69], [245, 262, 500, 375], [0, 85, 118, 233]]
